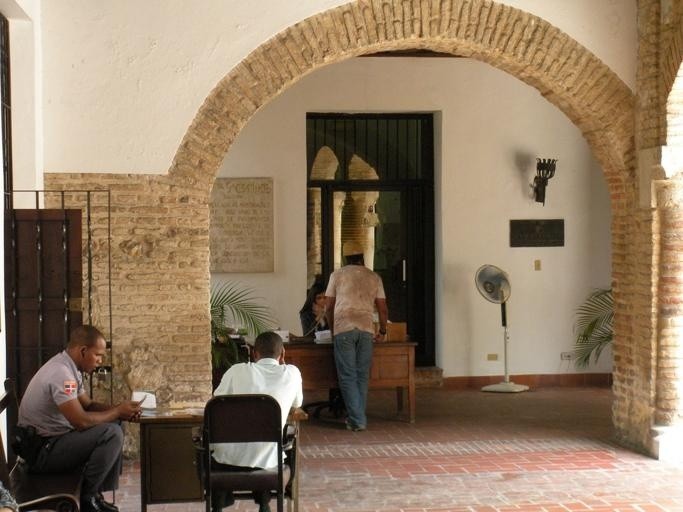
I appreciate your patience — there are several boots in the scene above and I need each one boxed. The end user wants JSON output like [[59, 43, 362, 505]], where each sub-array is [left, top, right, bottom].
[[79, 492, 118, 512]]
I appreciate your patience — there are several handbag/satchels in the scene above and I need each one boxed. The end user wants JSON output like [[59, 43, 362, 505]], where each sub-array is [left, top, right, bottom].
[[10, 424, 46, 467]]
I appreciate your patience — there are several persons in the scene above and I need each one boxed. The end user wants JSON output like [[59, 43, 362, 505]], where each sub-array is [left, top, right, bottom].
[[0, 481, 57, 512], [13, 324, 143, 512], [326, 239, 390, 433], [300, 281, 330, 336], [209, 330, 309, 512]]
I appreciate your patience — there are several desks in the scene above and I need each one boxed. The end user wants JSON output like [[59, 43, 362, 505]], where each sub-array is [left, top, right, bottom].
[[128, 404, 309, 512], [246, 338, 419, 424]]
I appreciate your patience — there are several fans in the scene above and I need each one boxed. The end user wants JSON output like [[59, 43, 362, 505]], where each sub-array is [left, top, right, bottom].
[[474, 265, 530, 393]]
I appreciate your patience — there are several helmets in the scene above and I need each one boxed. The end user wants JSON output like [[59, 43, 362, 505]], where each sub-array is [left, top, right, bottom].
[[342, 240, 364, 256]]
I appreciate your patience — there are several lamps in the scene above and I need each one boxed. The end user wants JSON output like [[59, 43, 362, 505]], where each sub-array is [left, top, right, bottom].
[[533, 157, 559, 206]]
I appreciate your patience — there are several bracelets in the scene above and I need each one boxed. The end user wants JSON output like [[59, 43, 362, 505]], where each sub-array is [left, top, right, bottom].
[[378, 329, 386, 334]]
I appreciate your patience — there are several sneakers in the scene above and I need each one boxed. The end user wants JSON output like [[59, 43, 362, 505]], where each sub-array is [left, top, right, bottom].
[[344, 419, 367, 432]]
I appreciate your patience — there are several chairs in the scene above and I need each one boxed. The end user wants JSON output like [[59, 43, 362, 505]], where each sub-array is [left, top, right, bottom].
[[1, 375, 87, 510], [192, 394, 298, 510]]
